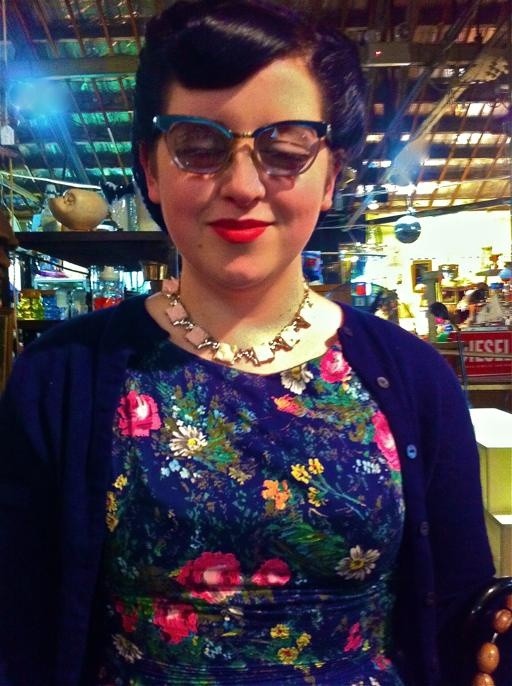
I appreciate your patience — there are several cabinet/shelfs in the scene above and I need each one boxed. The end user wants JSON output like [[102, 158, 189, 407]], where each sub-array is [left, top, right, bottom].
[[12, 224, 367, 348]]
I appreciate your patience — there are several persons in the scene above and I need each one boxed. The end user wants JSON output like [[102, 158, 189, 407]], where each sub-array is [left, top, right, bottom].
[[2, 0, 511, 683]]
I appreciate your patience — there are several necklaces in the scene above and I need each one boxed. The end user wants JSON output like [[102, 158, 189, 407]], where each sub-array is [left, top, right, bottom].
[[157, 272, 323, 366]]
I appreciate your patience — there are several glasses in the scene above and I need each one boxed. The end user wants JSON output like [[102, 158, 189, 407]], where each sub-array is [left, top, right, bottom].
[[151, 113, 332, 177]]
